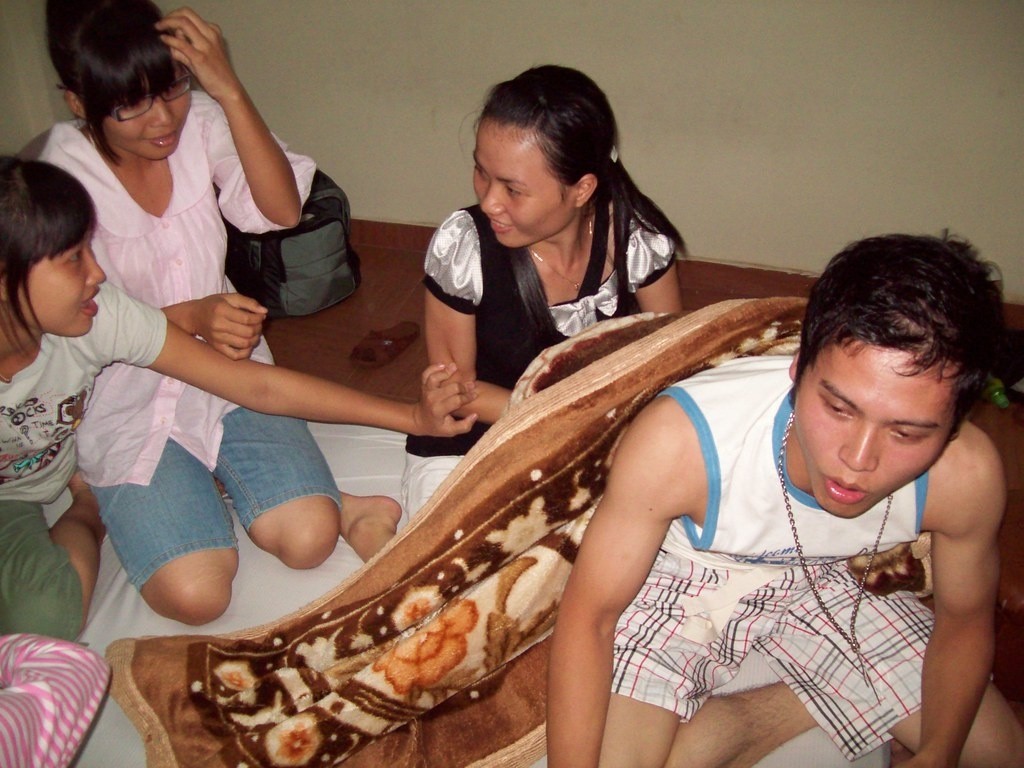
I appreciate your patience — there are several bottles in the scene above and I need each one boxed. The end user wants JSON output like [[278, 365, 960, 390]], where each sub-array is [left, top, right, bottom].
[[977, 372, 1010, 409]]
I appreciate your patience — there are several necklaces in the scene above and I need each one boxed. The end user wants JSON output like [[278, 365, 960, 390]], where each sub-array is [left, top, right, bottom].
[[0, 374, 11, 383], [778, 408, 894, 707], [529, 216, 593, 291]]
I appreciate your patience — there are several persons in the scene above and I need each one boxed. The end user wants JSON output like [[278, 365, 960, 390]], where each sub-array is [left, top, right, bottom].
[[0, 156, 479, 638], [34, 0, 340, 626], [339, 62, 689, 564], [544, 233, 1024, 768]]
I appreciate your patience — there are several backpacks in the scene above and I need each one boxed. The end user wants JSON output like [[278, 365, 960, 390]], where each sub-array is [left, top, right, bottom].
[[214, 168, 361, 316]]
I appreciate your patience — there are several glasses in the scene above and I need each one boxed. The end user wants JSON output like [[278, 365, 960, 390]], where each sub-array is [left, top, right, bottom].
[[55, 63, 190, 122]]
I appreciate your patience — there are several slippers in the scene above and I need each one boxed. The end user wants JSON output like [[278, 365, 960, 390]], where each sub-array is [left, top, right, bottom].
[[349, 321, 420, 367]]
